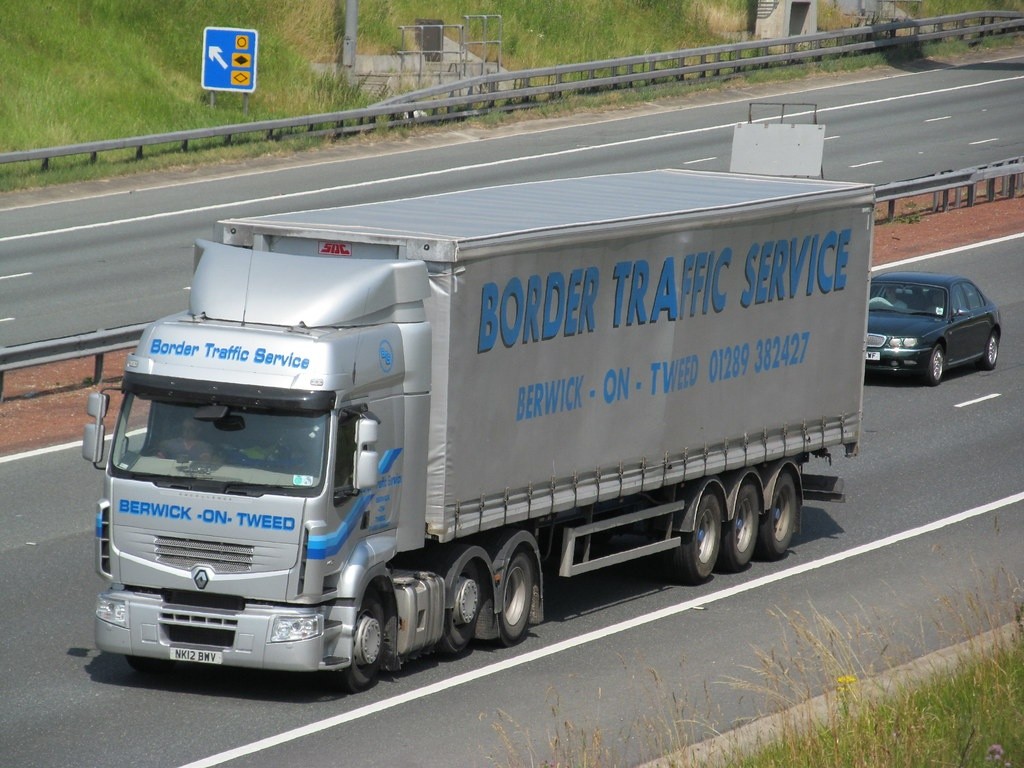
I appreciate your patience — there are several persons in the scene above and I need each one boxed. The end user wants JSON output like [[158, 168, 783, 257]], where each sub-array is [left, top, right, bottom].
[[244, 423, 313, 474], [150, 416, 214, 463], [884, 289, 907, 309]]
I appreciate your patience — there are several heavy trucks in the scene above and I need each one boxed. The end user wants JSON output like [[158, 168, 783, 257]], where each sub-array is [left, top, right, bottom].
[[80, 103, 878, 696]]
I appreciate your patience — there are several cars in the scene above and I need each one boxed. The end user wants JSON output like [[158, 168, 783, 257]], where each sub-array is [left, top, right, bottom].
[[863, 272, 1002, 384]]
